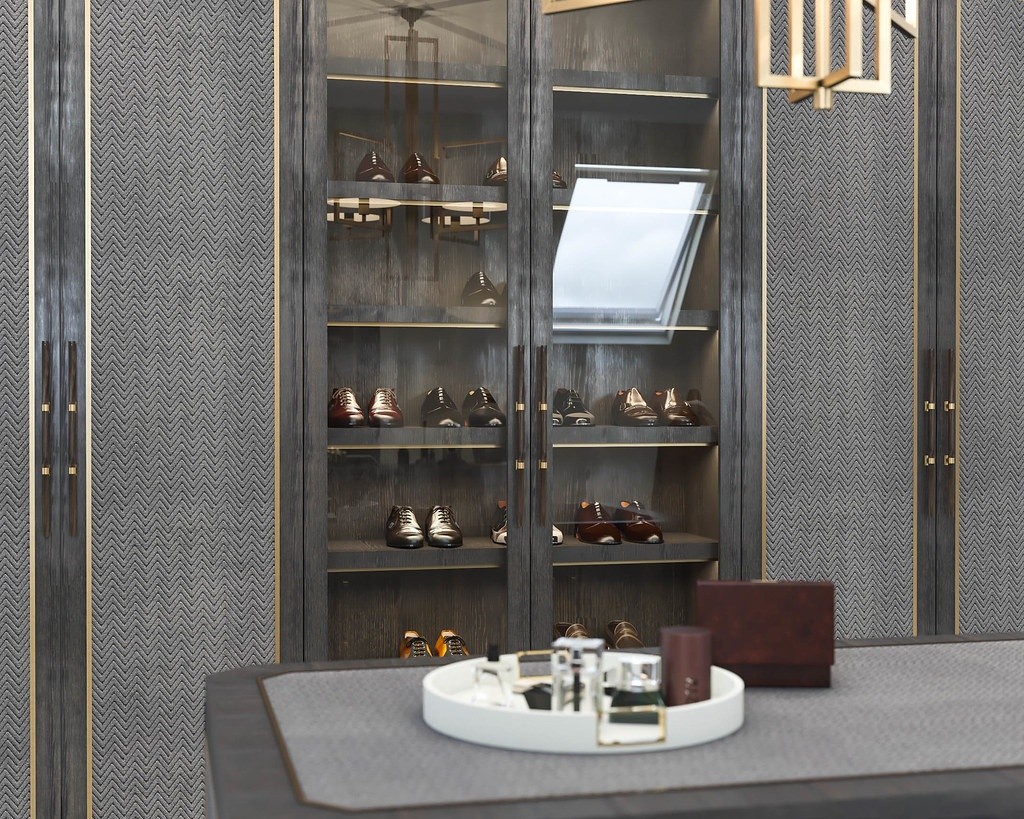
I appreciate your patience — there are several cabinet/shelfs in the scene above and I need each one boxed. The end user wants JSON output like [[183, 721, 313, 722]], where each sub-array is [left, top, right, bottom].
[[303, 0, 744, 664]]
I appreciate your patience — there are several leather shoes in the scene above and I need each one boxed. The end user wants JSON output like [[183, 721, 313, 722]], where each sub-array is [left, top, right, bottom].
[[552, 388, 596, 426], [396, 629, 433, 658], [483, 155, 509, 186], [463, 386, 507, 426], [613, 499, 665, 544], [553, 619, 590, 638], [551, 523, 565, 545], [462, 271, 501, 305], [491, 499, 509, 544], [385, 505, 424, 550], [573, 500, 622, 545], [612, 387, 659, 427], [651, 389, 697, 426], [433, 630, 469, 657], [425, 503, 463, 548], [355, 152, 394, 182], [421, 387, 463, 426], [330, 387, 365, 426], [402, 152, 440, 183], [367, 385, 404, 428], [605, 618, 644, 649], [554, 168, 567, 189]]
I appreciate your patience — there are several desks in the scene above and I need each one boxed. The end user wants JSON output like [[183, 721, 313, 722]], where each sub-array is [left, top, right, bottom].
[[203, 632, 1024, 819]]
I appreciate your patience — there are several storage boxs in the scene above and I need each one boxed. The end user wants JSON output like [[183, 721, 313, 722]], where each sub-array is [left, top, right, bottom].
[[689, 581, 836, 692]]
